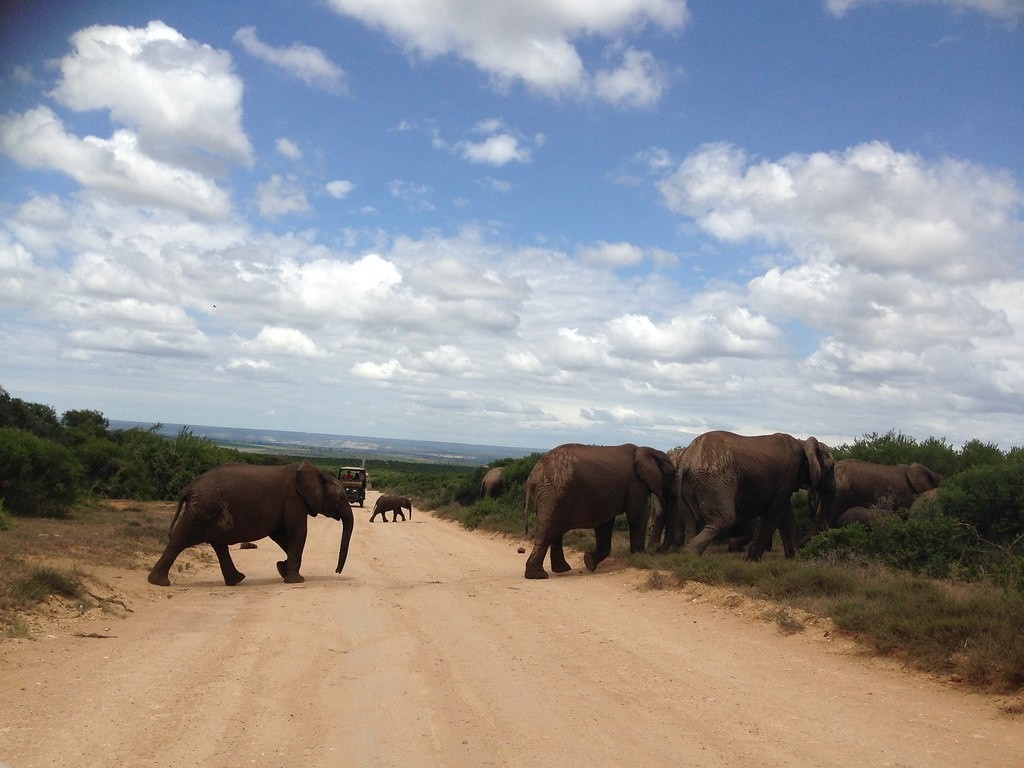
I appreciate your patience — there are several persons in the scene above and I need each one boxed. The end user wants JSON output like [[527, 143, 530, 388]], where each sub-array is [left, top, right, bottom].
[[351, 473, 361, 482], [343, 470, 352, 479]]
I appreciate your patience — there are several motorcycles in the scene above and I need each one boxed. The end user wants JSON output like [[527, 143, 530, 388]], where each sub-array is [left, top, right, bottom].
[[338, 466, 368, 508]]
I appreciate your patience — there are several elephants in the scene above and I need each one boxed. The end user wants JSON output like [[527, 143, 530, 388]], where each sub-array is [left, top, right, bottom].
[[369, 496, 411, 523], [477, 430, 947, 579], [147, 460, 354, 587]]
